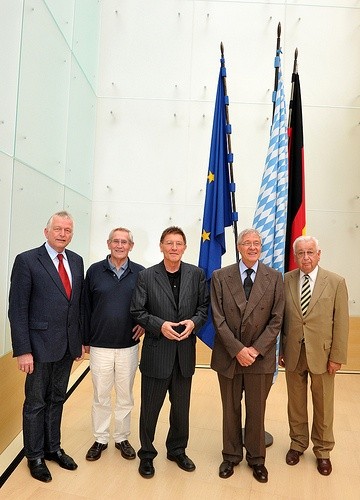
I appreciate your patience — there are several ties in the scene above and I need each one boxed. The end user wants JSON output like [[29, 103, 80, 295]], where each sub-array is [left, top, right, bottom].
[[57, 254, 71, 301], [243, 269, 254, 300], [301, 275, 311, 343]]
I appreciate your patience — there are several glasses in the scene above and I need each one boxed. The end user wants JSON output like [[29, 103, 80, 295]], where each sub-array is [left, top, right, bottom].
[[241, 241, 262, 247]]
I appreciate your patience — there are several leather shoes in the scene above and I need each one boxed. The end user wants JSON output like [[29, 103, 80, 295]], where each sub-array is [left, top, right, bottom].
[[167, 453, 195, 471], [286, 449, 303, 465], [115, 440, 136, 459], [317, 458, 332, 475], [85, 442, 108, 460], [28, 458, 51, 482], [46, 448, 77, 470], [248, 462, 268, 482], [219, 460, 239, 478], [139, 458, 153, 477]]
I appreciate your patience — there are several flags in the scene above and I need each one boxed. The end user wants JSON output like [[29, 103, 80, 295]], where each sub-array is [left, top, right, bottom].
[[195, 58, 238, 351], [252, 60, 307, 384]]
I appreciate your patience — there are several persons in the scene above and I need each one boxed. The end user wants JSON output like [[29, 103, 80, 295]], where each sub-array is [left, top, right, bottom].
[[9, 212, 84, 482], [278, 236, 350, 475], [130, 226, 210, 479], [81, 227, 145, 461], [210, 227, 286, 482]]
